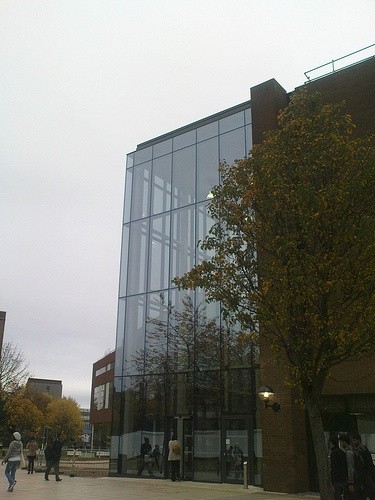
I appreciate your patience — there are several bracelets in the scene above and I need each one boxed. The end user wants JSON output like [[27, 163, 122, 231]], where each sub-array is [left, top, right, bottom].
[[2, 462, 3, 463]]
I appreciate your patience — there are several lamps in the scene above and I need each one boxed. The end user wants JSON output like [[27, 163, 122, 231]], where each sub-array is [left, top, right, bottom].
[[257, 386, 282, 412]]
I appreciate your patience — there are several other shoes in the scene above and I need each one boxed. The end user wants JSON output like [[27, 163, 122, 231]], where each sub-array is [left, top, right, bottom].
[[45, 477, 49, 480], [8, 482, 16, 491], [56, 477, 62, 481]]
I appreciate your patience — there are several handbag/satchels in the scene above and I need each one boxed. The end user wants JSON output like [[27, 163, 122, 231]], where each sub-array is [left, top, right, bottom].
[[19, 454, 25, 468], [44, 448, 52, 460], [174, 440, 181, 455]]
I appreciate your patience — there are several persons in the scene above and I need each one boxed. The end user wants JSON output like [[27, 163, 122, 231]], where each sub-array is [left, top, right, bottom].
[[0, 431, 24, 492], [167, 435, 183, 481], [137, 436, 163, 476], [328, 433, 375, 500], [25, 436, 39, 474], [44, 434, 64, 482], [216, 442, 246, 478]]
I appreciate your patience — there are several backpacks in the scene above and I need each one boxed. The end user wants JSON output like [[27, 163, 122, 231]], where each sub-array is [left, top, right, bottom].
[[345, 448, 367, 483]]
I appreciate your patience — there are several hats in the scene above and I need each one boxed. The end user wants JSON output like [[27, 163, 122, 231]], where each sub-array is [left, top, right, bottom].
[[13, 432, 21, 440], [329, 435, 340, 441]]
